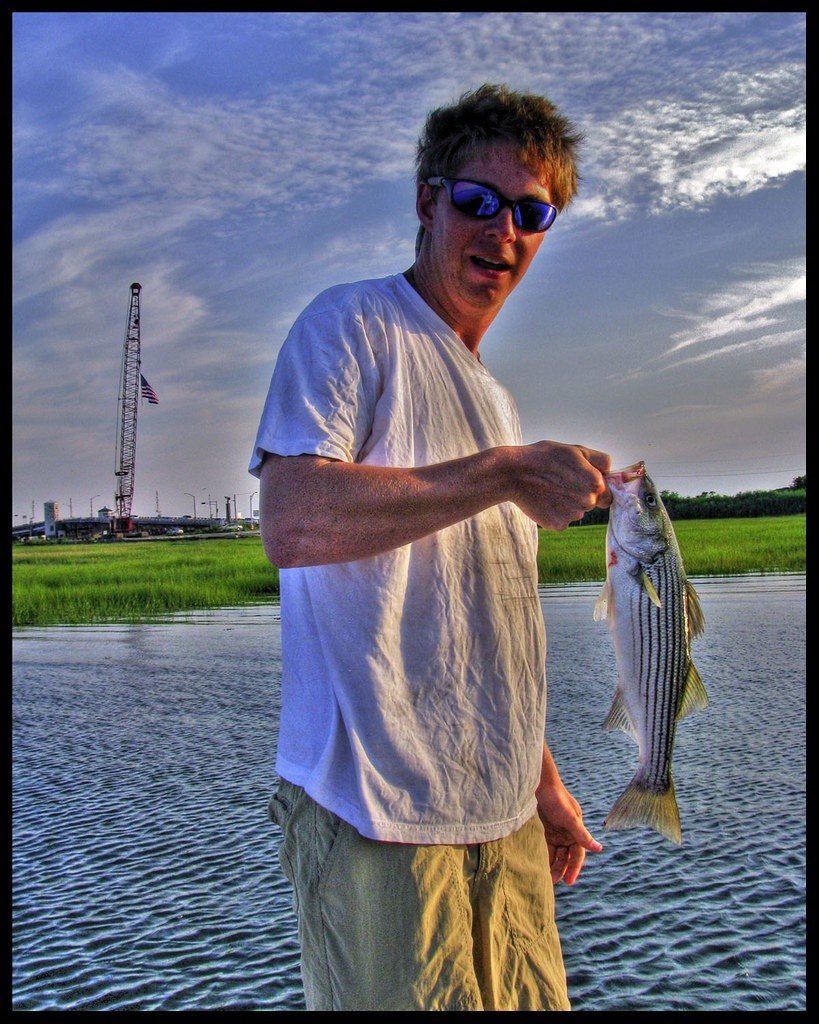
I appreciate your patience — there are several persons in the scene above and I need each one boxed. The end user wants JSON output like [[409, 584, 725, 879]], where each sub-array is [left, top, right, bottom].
[[247, 84, 613, 1011]]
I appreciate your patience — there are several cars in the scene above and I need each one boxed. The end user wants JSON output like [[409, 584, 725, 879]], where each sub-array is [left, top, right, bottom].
[[217, 523, 243, 533], [165, 528, 184, 537]]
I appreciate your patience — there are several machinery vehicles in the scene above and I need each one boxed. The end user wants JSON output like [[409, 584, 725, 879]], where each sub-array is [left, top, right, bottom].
[[110, 281, 144, 534]]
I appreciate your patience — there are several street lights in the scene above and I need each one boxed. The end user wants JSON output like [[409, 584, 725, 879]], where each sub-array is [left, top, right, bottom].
[[250, 491, 257, 520], [90, 495, 100, 517], [184, 492, 198, 521]]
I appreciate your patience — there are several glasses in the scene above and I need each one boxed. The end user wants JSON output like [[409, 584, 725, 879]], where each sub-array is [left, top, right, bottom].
[[426, 176, 558, 233]]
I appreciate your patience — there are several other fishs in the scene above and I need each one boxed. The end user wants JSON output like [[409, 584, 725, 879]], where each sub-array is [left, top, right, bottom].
[[593, 461, 710, 847]]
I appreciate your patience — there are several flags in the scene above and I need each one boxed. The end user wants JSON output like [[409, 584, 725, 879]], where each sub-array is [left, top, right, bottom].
[[141, 373, 160, 405]]
